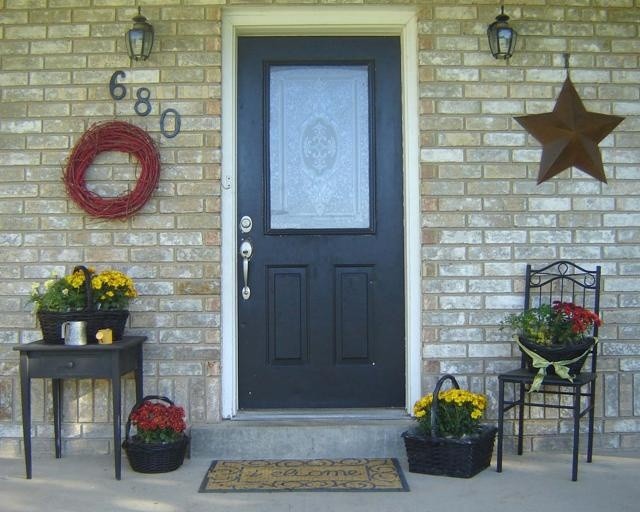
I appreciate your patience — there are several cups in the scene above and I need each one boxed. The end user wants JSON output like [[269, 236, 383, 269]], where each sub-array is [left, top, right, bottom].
[[59, 320, 88, 346]]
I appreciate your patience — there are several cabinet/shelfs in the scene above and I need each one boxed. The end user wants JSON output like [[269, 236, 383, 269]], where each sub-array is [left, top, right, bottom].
[[12, 335, 147, 480]]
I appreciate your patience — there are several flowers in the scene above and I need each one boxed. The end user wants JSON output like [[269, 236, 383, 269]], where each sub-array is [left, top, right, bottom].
[[29, 267, 139, 315], [130, 401, 186, 445], [498, 300, 601, 348], [413, 389, 488, 440]]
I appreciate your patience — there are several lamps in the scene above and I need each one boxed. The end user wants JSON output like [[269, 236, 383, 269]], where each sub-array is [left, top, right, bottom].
[[124, 5, 154, 62], [487, 5, 518, 60]]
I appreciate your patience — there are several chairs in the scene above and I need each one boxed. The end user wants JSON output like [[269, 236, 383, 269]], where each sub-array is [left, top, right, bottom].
[[497, 259, 601, 481]]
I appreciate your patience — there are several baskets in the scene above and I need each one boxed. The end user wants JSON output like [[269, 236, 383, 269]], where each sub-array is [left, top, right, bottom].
[[35, 267, 127, 345], [516, 335, 595, 374], [400, 372, 498, 479], [119, 396, 190, 474]]
